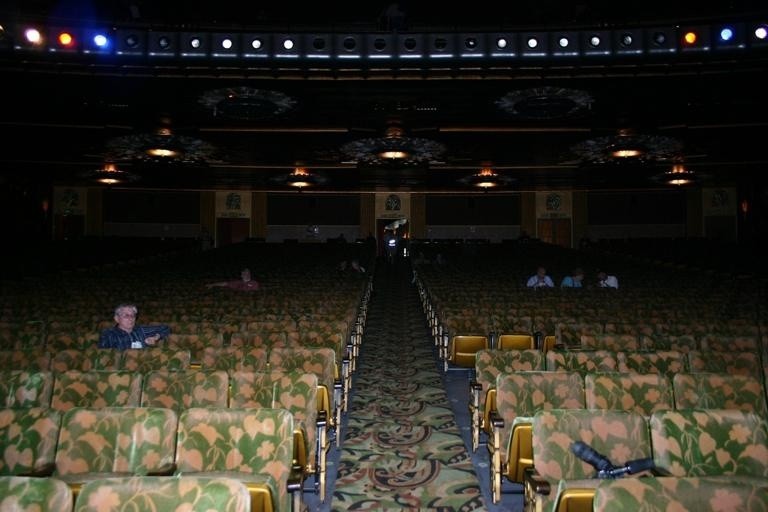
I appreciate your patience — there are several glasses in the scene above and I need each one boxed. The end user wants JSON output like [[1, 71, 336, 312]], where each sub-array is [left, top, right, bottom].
[[116, 312, 136, 318]]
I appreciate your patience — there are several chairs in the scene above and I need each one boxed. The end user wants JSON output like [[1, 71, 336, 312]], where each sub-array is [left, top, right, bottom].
[[416, 266, 767, 512], [1, 270, 372, 512]]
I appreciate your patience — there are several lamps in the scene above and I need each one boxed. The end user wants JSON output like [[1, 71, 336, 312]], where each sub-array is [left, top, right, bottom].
[[110, 117, 216, 170], [86, 158, 141, 187], [640, 156, 711, 188], [339, 123, 446, 171], [460, 165, 514, 189], [270, 165, 330, 192], [575, 130, 684, 168]]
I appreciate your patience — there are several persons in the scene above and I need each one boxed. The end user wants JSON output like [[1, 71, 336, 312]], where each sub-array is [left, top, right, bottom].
[[599, 273, 619, 289], [526, 267, 555, 287], [560, 268, 585, 287], [99, 303, 171, 347], [204, 268, 259, 292]]
